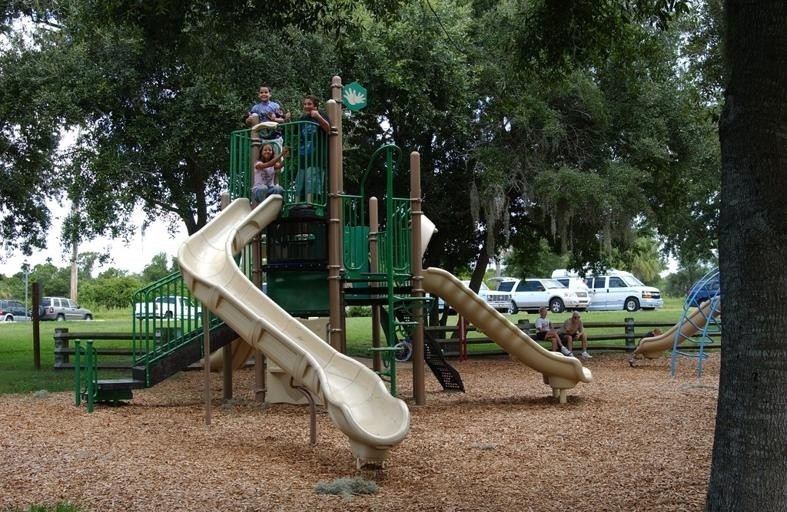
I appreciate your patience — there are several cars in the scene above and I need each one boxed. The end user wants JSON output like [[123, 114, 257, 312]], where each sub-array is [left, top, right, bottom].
[[686, 279, 720, 308], [486, 276, 580, 316], [423, 279, 512, 316]]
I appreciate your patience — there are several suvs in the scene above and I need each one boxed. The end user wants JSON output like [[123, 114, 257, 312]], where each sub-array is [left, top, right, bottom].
[[0, 299, 33, 322], [30, 296, 94, 322]]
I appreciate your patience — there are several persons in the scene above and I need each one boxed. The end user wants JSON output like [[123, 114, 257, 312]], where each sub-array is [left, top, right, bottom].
[[245, 84, 289, 185], [252, 143, 290, 203], [557, 311, 592, 359], [534, 307, 571, 356], [294, 95, 330, 204]]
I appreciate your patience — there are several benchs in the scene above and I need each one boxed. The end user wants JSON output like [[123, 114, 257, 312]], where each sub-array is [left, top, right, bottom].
[[410, 321, 721, 356], [50, 327, 174, 373]]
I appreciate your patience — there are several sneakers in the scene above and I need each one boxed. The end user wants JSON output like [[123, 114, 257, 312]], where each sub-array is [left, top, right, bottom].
[[562, 346, 592, 359]]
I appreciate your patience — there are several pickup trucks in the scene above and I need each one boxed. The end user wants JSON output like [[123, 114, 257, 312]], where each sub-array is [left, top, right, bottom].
[[135, 295, 202, 319]]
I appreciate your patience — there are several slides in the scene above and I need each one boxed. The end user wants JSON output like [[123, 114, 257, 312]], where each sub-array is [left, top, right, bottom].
[[633, 296, 721, 367], [200, 338, 254, 373], [423, 267, 592, 403], [178, 193, 411, 460]]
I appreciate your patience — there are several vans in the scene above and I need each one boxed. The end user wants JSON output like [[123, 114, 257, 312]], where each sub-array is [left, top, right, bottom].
[[580, 272, 663, 312], [557, 276, 592, 312]]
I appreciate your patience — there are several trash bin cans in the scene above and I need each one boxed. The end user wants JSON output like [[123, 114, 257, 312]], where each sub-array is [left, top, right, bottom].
[[154, 327, 183, 348]]
